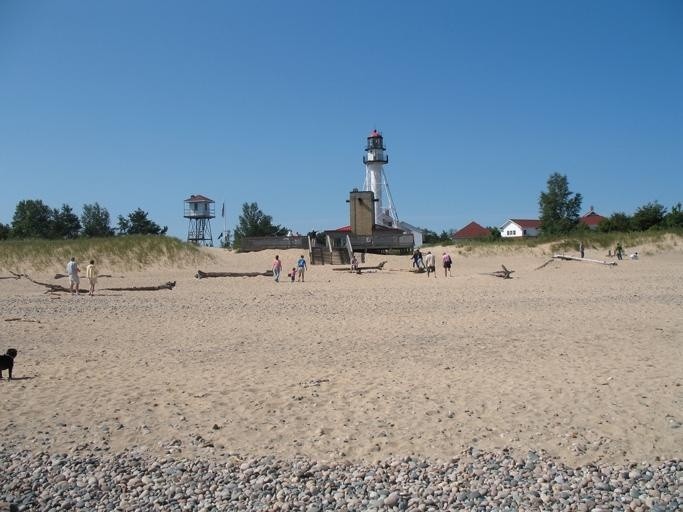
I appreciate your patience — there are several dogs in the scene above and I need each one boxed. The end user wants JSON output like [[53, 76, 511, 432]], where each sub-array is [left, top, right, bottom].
[[0, 348, 17, 380]]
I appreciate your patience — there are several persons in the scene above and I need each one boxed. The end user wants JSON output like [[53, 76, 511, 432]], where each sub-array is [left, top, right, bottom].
[[579, 240, 586, 258], [310, 227, 320, 247], [272, 254, 283, 282], [615, 242, 624, 260], [629, 252, 639, 259], [85, 259, 97, 296], [411, 249, 452, 277], [65, 256, 83, 295], [289, 268, 297, 281], [350, 255, 359, 270], [297, 256, 308, 282], [371, 128, 379, 136]]
